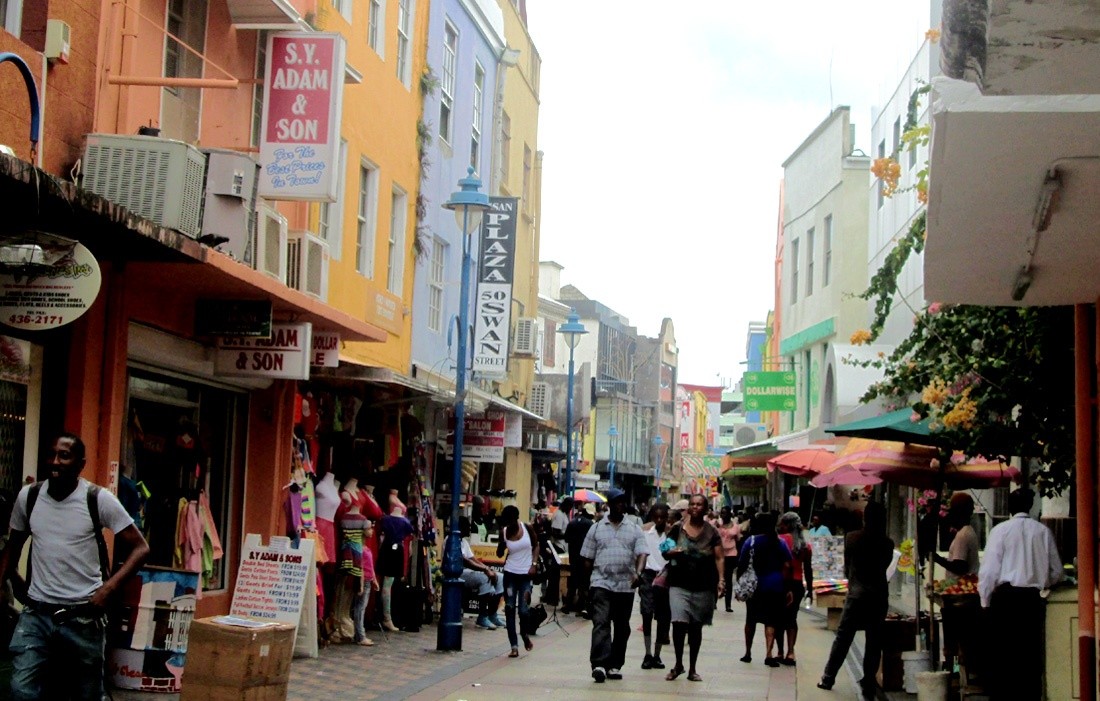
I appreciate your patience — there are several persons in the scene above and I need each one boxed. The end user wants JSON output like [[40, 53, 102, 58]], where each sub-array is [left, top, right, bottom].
[[312, 471, 409, 644], [978, 486, 1065, 701], [1, 433, 152, 701], [443, 491, 795, 682]]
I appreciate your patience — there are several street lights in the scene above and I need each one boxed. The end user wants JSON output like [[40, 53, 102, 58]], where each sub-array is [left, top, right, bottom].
[[605, 425, 619, 490], [554, 305, 590, 495], [436, 163, 500, 651], [651, 435, 664, 505]]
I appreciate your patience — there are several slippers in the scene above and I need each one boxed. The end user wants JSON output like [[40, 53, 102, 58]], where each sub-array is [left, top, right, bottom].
[[666, 670, 685, 681], [688, 671, 701, 682], [740, 655, 795, 668]]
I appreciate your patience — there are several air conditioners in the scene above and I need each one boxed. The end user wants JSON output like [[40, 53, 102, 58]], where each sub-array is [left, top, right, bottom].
[[287, 229, 330, 303], [83, 132, 207, 238], [733, 421, 767, 448], [246, 202, 288, 287], [195, 146, 262, 271], [513, 317, 539, 356], [531, 380, 552, 419]]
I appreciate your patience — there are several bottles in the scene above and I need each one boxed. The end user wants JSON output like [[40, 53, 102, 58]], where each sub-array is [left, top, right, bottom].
[[921, 628, 926, 653], [660, 537, 677, 565]]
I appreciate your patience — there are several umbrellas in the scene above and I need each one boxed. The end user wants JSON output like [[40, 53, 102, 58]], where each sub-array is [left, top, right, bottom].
[[556, 487, 608, 506], [767, 402, 1025, 701]]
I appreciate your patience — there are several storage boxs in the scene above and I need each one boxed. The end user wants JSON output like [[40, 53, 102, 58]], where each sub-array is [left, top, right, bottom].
[[825, 606, 844, 629], [179, 614, 297, 701], [817, 584, 850, 608], [877, 612, 939, 691]]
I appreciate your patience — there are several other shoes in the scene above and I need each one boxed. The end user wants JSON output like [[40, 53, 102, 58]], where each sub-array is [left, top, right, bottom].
[[509, 651, 518, 658], [607, 668, 622, 680], [860, 689, 876, 700], [817, 681, 832, 691], [592, 667, 606, 683], [524, 641, 533, 651]]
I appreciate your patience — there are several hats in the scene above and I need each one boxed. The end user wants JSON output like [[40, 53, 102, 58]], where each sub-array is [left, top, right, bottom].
[[606, 488, 624, 500]]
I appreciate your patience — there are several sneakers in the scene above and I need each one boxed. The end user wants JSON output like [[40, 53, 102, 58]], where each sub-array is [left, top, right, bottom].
[[489, 613, 506, 626], [476, 615, 496, 630]]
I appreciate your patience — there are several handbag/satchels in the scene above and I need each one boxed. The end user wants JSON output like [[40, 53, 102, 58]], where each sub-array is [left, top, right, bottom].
[[651, 564, 672, 594], [735, 565, 758, 602]]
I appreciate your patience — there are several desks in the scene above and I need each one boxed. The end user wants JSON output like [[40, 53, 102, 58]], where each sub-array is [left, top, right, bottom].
[[926, 572, 991, 695]]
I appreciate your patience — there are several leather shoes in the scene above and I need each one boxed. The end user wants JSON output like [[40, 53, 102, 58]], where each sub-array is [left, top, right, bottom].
[[652, 658, 665, 669], [642, 657, 653, 669]]
